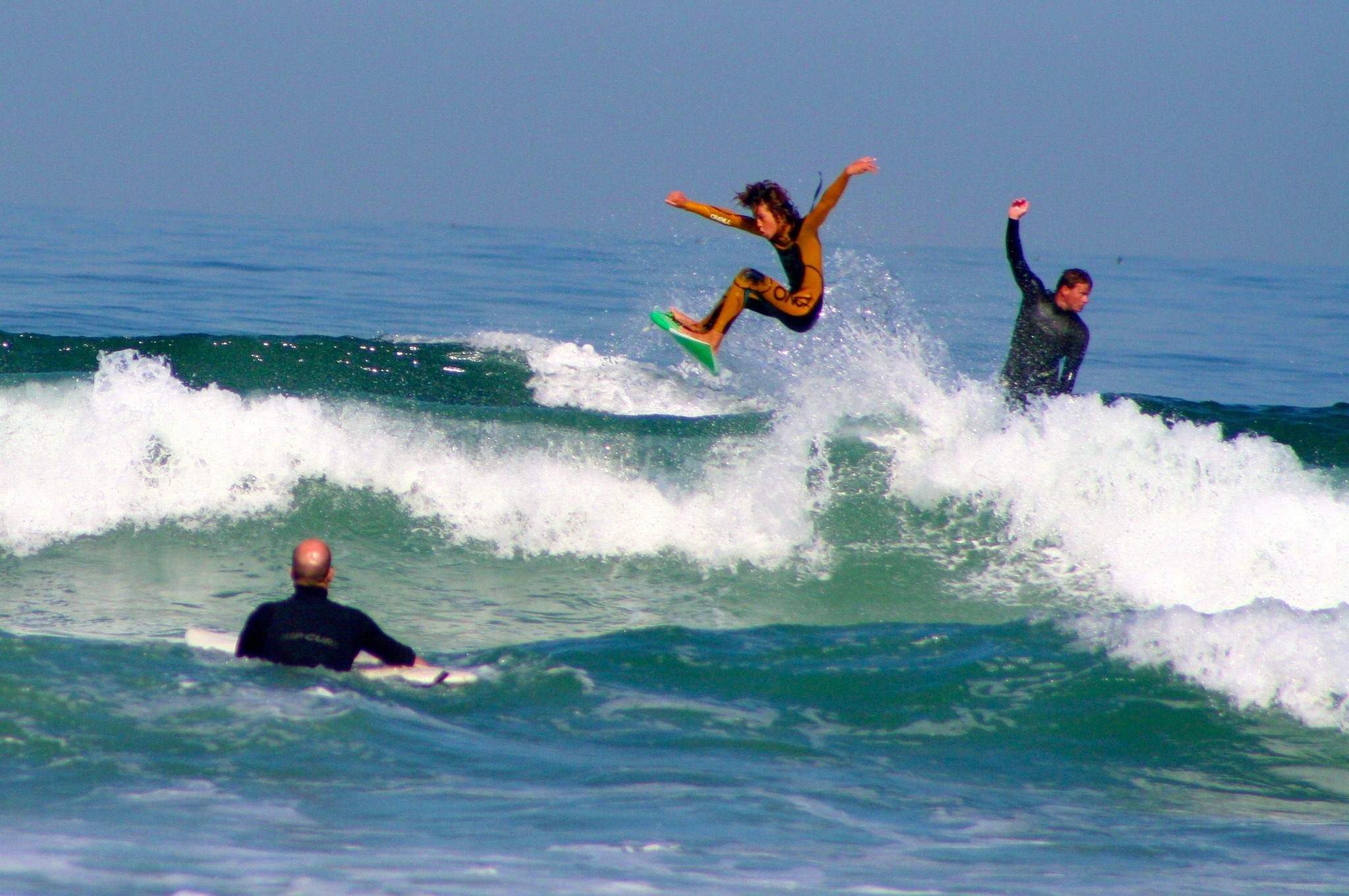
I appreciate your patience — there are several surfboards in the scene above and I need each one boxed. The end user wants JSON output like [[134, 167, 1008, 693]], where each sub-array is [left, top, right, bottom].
[[184, 628, 478, 685], [650, 305, 721, 379]]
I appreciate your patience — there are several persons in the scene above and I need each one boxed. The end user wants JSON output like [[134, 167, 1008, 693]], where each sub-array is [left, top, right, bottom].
[[234, 539, 432, 671], [665, 156, 880, 353], [1005, 197, 1092, 397]]
